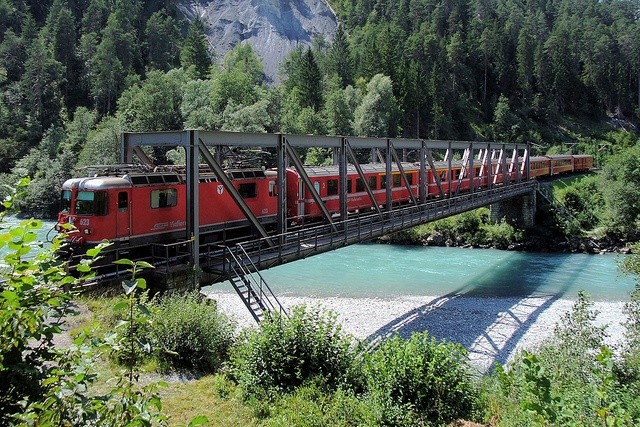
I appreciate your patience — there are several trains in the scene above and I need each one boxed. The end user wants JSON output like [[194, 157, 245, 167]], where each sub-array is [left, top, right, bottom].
[[62, 155, 595, 253]]
